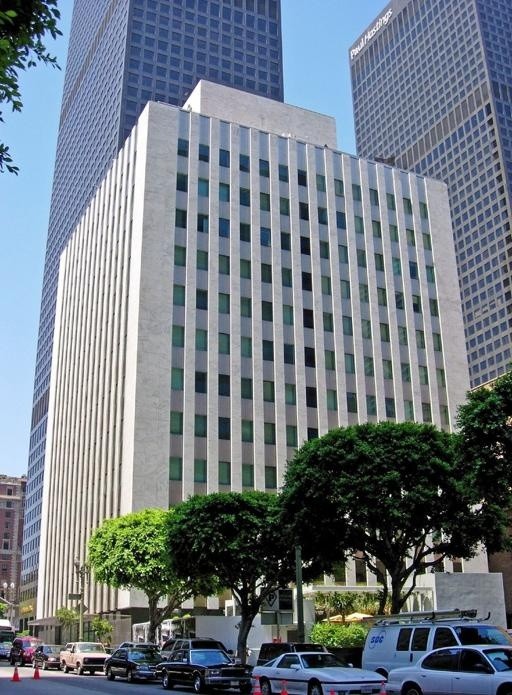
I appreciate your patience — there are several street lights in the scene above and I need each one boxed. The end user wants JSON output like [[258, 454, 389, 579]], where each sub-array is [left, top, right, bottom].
[[4, 582, 16, 623], [74, 553, 92, 641]]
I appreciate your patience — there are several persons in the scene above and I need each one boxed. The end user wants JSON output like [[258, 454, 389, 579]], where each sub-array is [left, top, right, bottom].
[[235, 644, 251, 665]]
